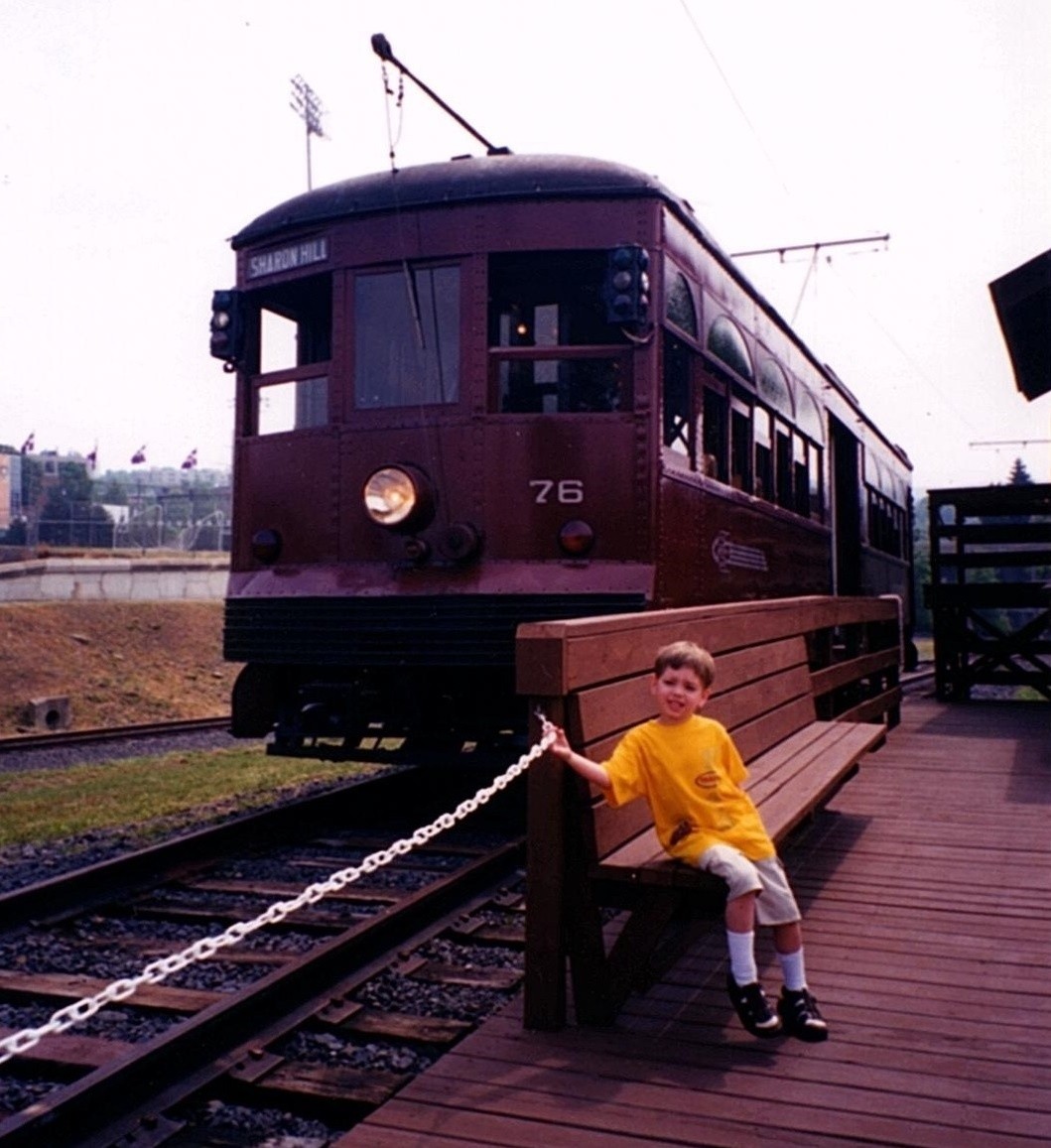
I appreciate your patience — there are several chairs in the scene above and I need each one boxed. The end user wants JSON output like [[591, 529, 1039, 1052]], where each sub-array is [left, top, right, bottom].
[[704, 454, 765, 498]]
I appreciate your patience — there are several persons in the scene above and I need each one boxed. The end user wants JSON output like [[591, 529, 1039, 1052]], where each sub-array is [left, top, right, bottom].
[[540, 640, 829, 1045]]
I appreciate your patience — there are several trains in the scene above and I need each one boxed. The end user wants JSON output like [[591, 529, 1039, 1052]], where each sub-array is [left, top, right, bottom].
[[203, 32, 912, 789]]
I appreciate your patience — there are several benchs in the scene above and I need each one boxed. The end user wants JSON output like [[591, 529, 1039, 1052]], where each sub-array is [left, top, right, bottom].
[[569, 636, 889, 1029]]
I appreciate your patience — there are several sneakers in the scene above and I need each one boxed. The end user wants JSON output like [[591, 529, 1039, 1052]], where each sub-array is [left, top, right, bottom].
[[726, 970, 784, 1039], [776, 984, 831, 1042]]
